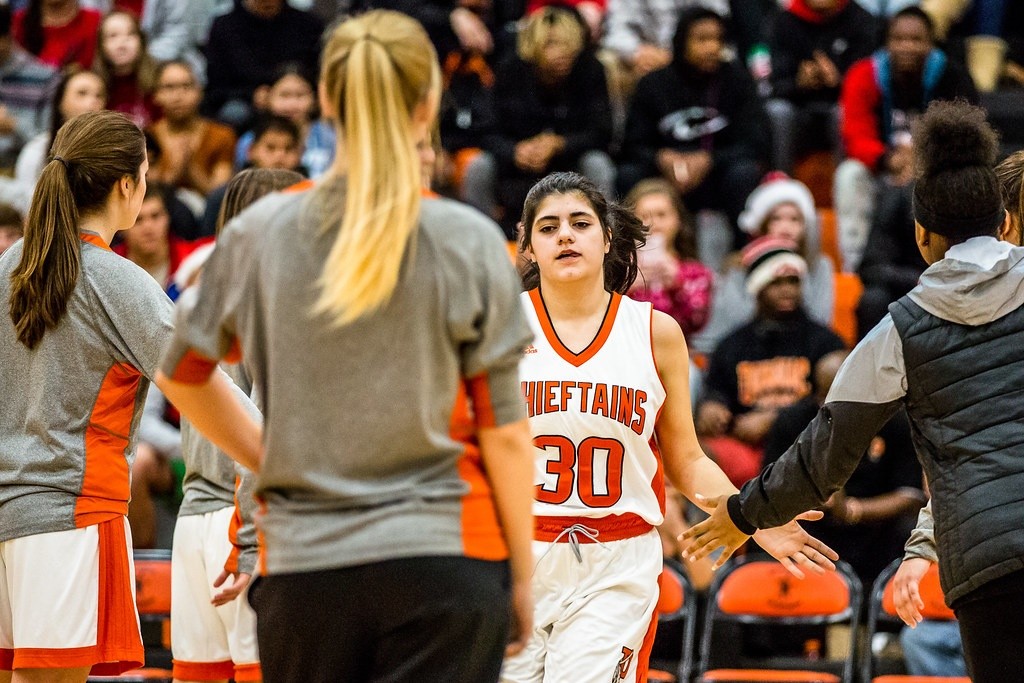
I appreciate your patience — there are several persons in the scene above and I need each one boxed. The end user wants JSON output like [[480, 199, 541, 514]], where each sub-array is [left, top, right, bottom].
[[0, 0, 1024, 683]]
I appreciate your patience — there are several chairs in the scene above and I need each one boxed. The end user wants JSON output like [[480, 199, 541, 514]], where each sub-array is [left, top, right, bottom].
[[643, 557, 973, 683], [87, 548, 175, 682]]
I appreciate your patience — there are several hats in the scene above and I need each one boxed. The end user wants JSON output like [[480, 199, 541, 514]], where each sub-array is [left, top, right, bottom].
[[740, 237, 809, 295], [738, 170, 819, 236]]
